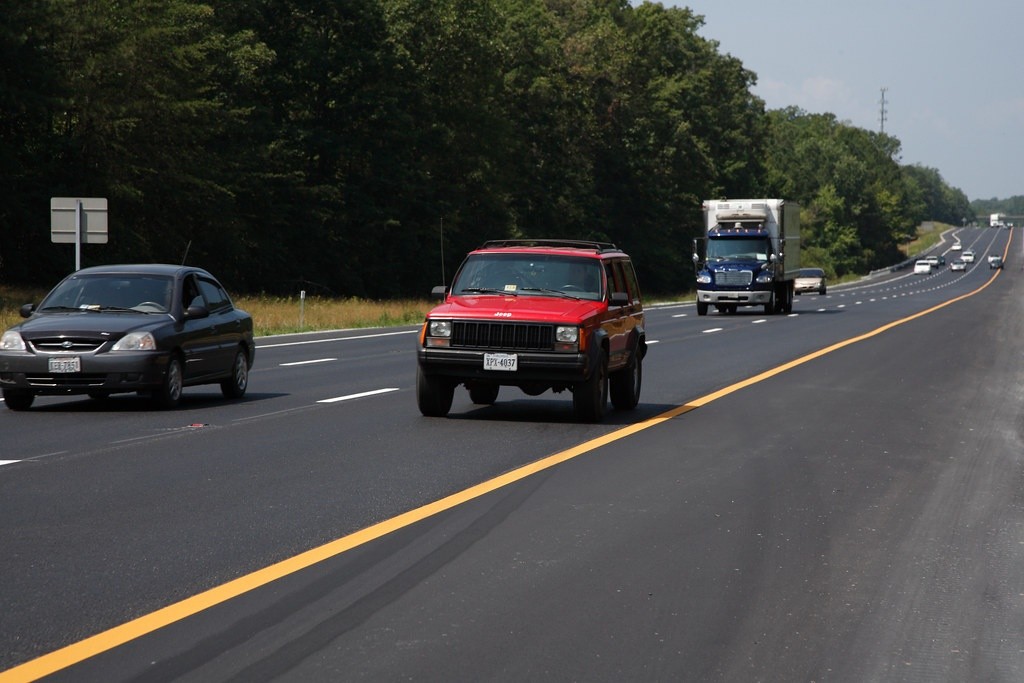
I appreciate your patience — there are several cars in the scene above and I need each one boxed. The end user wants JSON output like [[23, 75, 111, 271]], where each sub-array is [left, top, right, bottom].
[[914, 260, 931, 276], [950, 258, 967, 272], [0, 265, 255, 415], [959, 251, 977, 264], [950, 242, 964, 252], [987, 254, 1005, 270], [937, 255, 947, 266]]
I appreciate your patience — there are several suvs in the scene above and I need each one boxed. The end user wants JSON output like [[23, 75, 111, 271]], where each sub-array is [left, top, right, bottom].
[[925, 255, 941, 270], [415, 237, 647, 418], [795, 267, 828, 295]]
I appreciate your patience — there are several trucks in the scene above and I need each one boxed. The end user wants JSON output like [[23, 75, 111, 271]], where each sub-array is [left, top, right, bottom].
[[989, 213, 1003, 228], [690, 199, 801, 316]]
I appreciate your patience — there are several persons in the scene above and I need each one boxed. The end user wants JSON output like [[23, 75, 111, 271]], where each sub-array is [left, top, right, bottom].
[[568, 261, 588, 292]]
